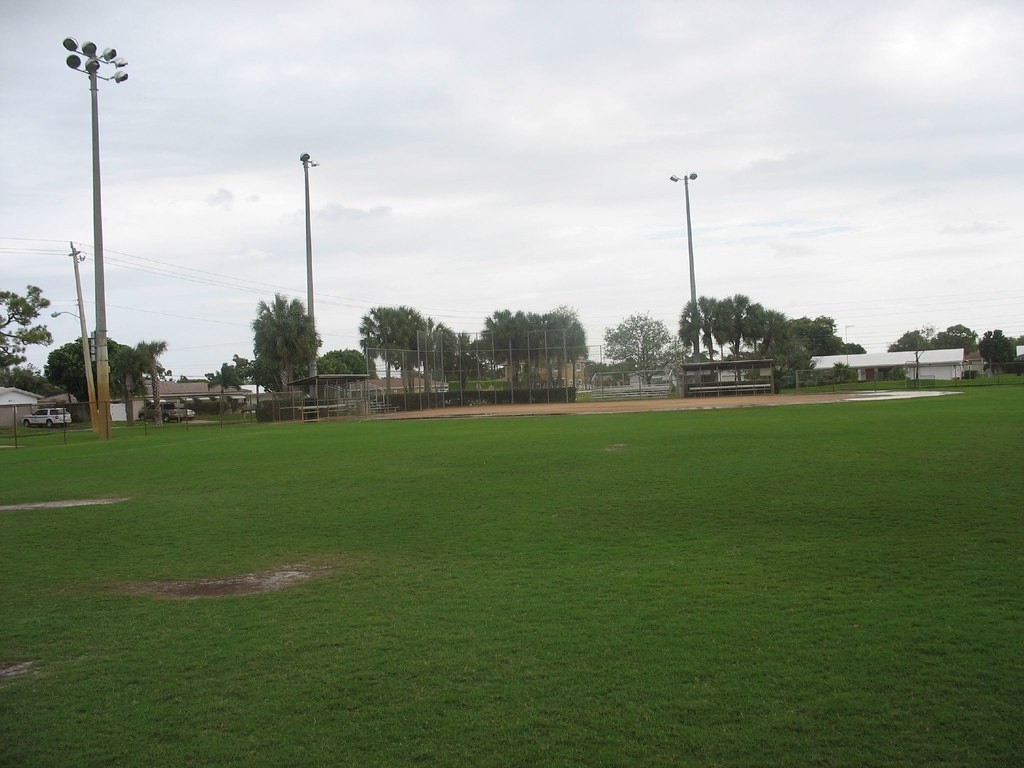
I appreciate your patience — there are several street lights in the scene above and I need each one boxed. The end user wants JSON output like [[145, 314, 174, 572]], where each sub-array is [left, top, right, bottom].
[[300, 152, 320, 419], [64, 35, 131, 442], [844, 324, 855, 367], [51, 310, 100, 433], [668, 171, 707, 398]]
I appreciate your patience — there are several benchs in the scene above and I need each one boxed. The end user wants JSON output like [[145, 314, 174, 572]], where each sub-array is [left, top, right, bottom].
[[302, 410, 320, 420]]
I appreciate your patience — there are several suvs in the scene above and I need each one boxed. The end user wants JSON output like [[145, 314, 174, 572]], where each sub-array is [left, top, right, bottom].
[[21, 407, 73, 428], [138, 401, 196, 422]]
